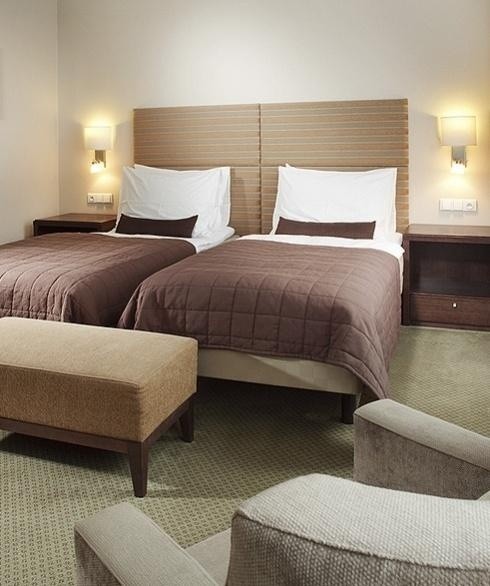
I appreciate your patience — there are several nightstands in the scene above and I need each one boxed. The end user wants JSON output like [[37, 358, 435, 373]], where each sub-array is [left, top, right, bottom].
[[29, 210, 120, 237], [401, 220, 489, 334]]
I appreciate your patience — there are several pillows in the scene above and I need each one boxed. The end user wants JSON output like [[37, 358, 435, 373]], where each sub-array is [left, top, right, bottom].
[[132, 162, 231, 228], [269, 165, 395, 242], [117, 165, 227, 237], [283, 160, 398, 235]]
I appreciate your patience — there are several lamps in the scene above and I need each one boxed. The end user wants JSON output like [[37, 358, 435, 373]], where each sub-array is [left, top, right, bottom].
[[79, 122, 116, 177], [437, 113, 478, 175]]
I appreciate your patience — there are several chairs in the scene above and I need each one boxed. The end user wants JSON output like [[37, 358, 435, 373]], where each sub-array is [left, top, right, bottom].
[[66, 392, 488, 585]]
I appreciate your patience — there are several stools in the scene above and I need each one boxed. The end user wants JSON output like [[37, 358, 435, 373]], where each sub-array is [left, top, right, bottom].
[[0, 308, 205, 501]]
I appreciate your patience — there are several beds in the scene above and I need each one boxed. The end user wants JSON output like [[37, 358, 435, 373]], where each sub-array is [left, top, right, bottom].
[[0, 98, 260, 327], [140, 96, 407, 428]]
